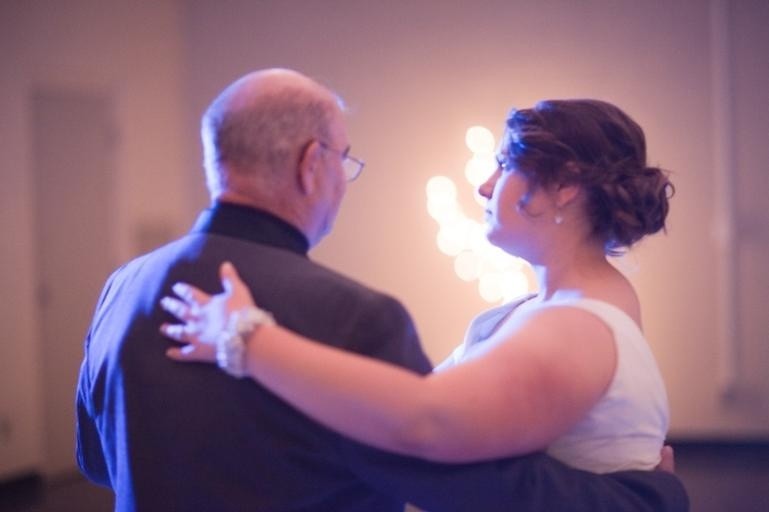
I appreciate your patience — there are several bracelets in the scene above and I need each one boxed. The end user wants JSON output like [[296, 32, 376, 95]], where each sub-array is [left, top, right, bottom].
[[215, 305, 274, 379]]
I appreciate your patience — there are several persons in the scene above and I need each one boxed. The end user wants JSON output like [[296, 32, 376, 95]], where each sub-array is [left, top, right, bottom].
[[156, 99, 670, 477], [75, 67, 690, 512]]
